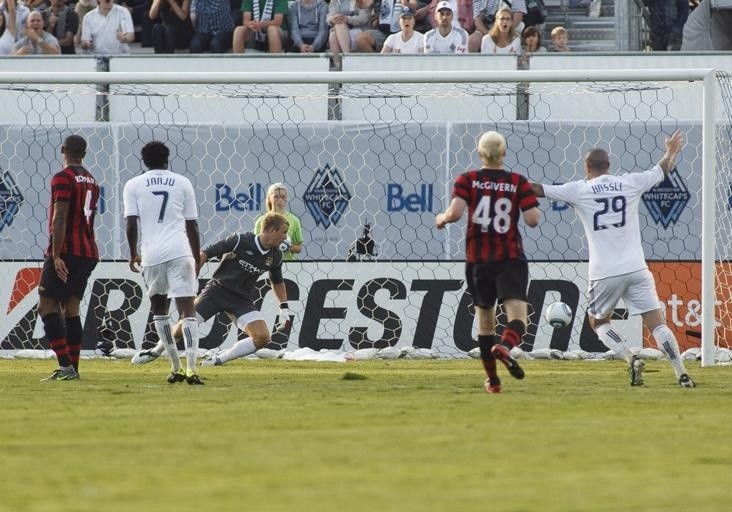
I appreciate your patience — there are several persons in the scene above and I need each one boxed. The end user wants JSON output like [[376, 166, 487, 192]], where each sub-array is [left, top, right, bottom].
[[549, 26, 571, 52], [633, 0, 702, 51], [131, 212, 291, 366], [124, 140, 207, 384], [130, 0, 236, 54], [528, 130, 697, 387], [0, 0, 135, 54], [253, 182, 303, 260], [40, 135, 100, 382], [433, 130, 539, 392], [234, 0, 548, 53]]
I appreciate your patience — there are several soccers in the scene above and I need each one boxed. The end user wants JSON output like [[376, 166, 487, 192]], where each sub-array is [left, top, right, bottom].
[[546, 302, 572, 329], [278, 234, 292, 252]]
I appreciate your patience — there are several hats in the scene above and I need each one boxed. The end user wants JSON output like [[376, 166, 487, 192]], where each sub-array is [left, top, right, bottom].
[[435, 0, 455, 14], [398, 6, 415, 18]]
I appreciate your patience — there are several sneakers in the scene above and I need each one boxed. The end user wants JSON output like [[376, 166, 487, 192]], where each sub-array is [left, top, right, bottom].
[[198, 354, 222, 367], [39, 366, 80, 382], [484, 377, 502, 394], [166, 367, 187, 385], [678, 373, 697, 388], [629, 354, 646, 387], [185, 369, 205, 386], [491, 343, 526, 380], [129, 347, 161, 366]]
